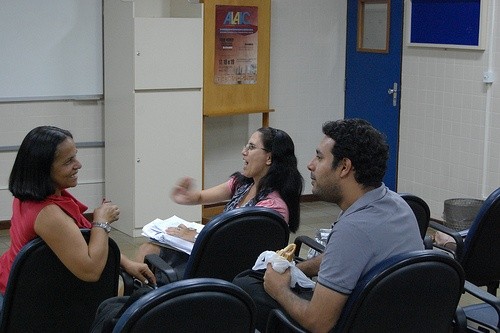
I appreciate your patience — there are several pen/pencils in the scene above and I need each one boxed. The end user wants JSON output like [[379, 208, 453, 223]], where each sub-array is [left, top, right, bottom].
[[147, 283, 159, 290]]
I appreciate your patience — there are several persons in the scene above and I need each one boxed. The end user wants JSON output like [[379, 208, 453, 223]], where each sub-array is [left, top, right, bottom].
[[0, 125, 157, 297], [137, 128, 302, 286], [263, 119, 425, 333]]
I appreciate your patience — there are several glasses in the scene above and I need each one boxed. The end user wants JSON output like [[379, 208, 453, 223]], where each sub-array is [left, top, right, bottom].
[[241, 144, 270, 153]]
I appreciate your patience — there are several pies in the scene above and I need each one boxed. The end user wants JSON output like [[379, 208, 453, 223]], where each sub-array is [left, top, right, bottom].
[[278, 243, 296, 262]]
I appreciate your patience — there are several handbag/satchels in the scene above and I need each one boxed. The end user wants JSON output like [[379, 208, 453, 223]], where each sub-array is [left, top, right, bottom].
[[252, 251, 316, 289]]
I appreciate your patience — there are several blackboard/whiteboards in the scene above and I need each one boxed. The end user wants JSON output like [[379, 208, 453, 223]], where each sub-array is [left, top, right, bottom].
[[0, 0, 105, 103]]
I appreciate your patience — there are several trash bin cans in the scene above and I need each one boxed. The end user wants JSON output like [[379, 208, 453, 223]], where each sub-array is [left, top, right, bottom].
[[445, 198, 484, 232]]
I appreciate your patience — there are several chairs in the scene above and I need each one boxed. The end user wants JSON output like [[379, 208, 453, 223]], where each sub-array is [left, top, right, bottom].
[[0, 187, 500, 333]]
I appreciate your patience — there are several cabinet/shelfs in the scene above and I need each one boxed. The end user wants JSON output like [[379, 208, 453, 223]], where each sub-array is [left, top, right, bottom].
[[103, 0, 205, 239]]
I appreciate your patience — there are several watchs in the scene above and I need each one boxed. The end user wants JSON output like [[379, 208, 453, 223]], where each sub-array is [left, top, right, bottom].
[[92, 222, 111, 233]]
